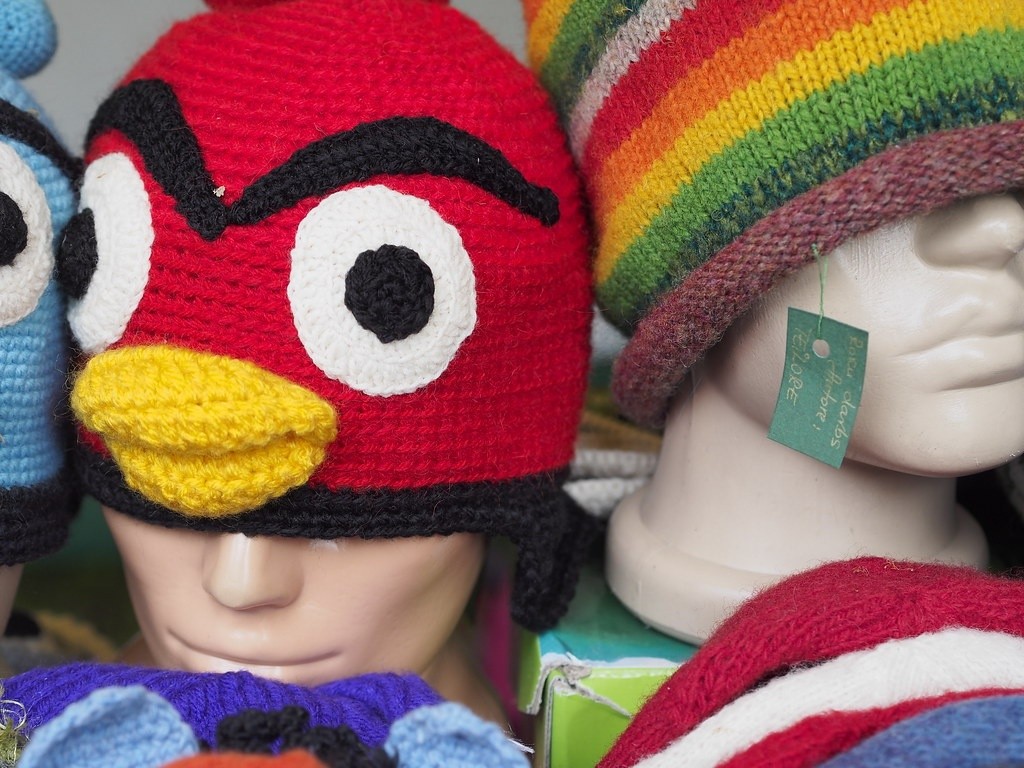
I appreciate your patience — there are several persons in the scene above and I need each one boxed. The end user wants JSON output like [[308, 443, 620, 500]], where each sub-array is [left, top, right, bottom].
[[517, 0, 1024, 647], [0, 1, 97, 656], [59, 1, 596, 764]]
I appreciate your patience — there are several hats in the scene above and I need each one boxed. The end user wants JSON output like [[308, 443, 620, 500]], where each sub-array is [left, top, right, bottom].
[[0, 1, 1023, 766]]
